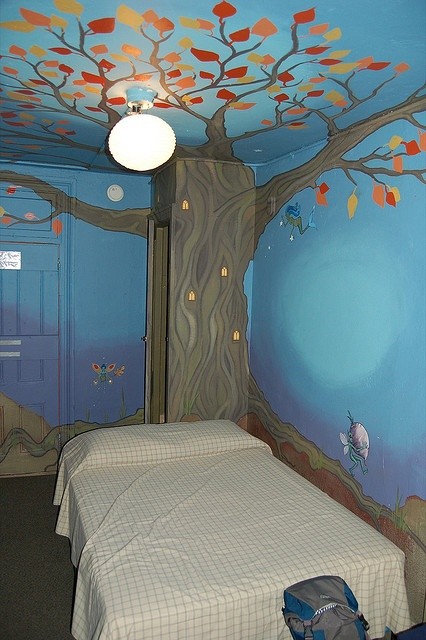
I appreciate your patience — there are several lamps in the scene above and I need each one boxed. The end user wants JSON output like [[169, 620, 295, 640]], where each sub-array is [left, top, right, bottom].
[[107, 86, 176, 172]]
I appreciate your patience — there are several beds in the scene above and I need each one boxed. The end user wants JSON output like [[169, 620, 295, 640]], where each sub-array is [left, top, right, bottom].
[[52, 418, 417, 640]]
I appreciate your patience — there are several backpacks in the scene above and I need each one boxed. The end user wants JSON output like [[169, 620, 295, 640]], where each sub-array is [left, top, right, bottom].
[[282, 576, 369, 640]]
